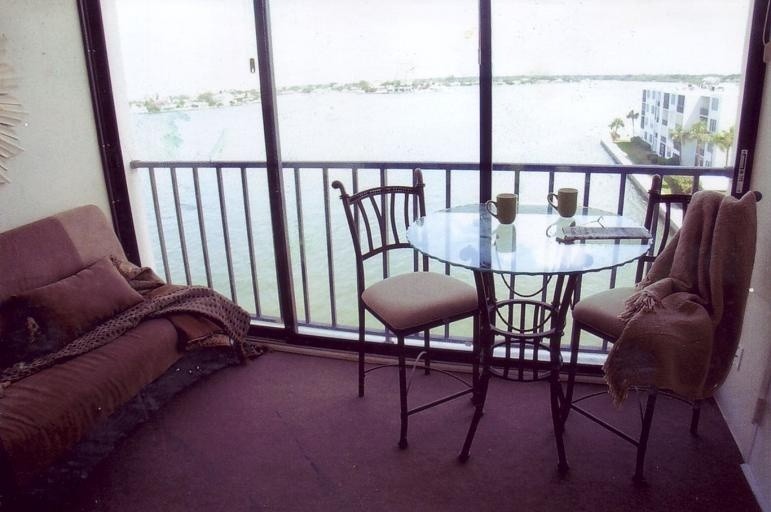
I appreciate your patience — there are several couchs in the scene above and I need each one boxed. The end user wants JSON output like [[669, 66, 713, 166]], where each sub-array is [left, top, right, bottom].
[[0, 197, 248, 510]]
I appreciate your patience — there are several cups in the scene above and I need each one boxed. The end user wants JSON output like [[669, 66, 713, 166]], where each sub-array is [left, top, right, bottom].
[[489, 223, 518, 253], [545, 217, 574, 246], [485, 192, 517, 225], [544, 187, 578, 217]]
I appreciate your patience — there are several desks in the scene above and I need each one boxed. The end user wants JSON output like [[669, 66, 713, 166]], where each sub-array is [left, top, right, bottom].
[[405, 200, 656, 473]]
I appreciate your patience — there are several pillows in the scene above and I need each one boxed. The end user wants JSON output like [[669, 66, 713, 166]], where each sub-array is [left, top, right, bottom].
[[18, 254, 145, 351]]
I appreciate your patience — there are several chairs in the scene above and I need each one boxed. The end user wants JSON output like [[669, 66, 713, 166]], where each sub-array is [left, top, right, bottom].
[[331, 169, 495, 448], [558, 171, 765, 483]]
